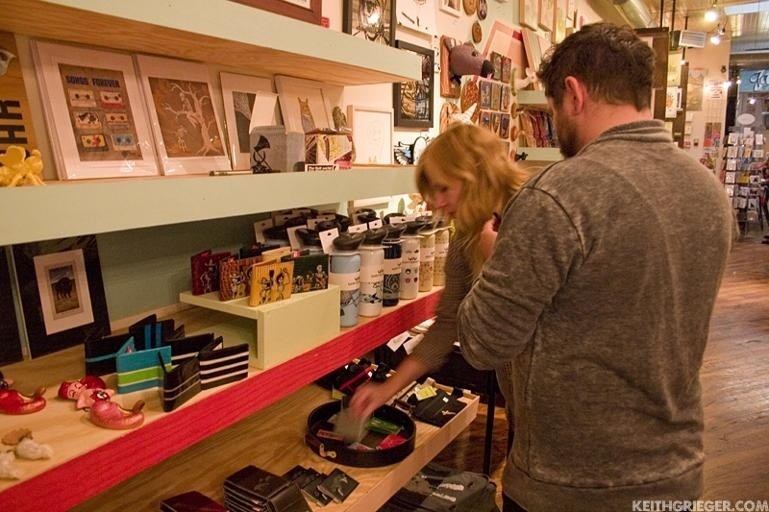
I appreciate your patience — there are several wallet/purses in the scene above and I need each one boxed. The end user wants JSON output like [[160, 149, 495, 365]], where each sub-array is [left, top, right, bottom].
[[190, 242, 330, 306], [85, 313, 250, 411]]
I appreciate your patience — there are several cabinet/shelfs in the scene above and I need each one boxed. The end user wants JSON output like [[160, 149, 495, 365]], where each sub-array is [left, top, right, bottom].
[[1, 0, 768, 512]]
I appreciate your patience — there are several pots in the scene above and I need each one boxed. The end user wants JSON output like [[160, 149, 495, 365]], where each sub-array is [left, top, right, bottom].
[[304, 397, 418, 469]]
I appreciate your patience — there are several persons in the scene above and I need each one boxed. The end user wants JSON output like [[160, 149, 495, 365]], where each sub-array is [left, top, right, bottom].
[[456, 21, 732, 511], [348, 125, 527, 418], [761, 153, 769, 244]]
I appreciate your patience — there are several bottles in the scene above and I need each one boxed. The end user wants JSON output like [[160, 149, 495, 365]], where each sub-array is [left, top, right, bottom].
[[329, 215, 452, 327]]
